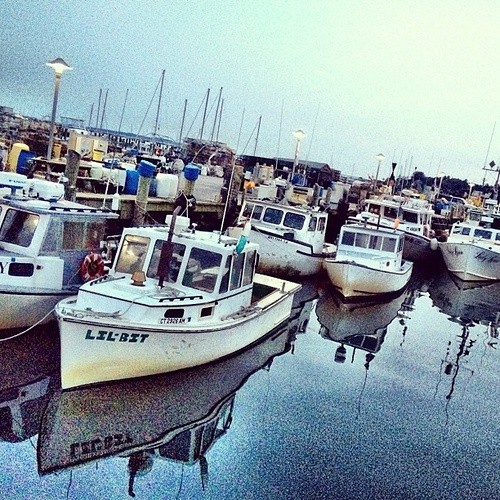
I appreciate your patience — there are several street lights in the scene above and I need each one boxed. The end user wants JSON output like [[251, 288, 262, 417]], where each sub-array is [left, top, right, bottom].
[[373, 153, 385, 191], [44, 57, 72, 171], [290, 129, 306, 182]]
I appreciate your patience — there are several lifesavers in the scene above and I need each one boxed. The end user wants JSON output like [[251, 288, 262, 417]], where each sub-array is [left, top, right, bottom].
[[80, 252, 105, 282]]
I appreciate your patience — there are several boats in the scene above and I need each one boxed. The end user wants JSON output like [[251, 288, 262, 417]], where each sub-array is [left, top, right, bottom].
[[323, 216, 415, 304], [54, 190, 303, 392], [222, 184, 337, 279], [0, 63, 500, 259], [36, 312, 310, 497], [436, 206, 500, 291], [0, 170, 120, 333], [315, 281, 423, 371], [0, 332, 61, 444], [427, 268, 500, 334]]
